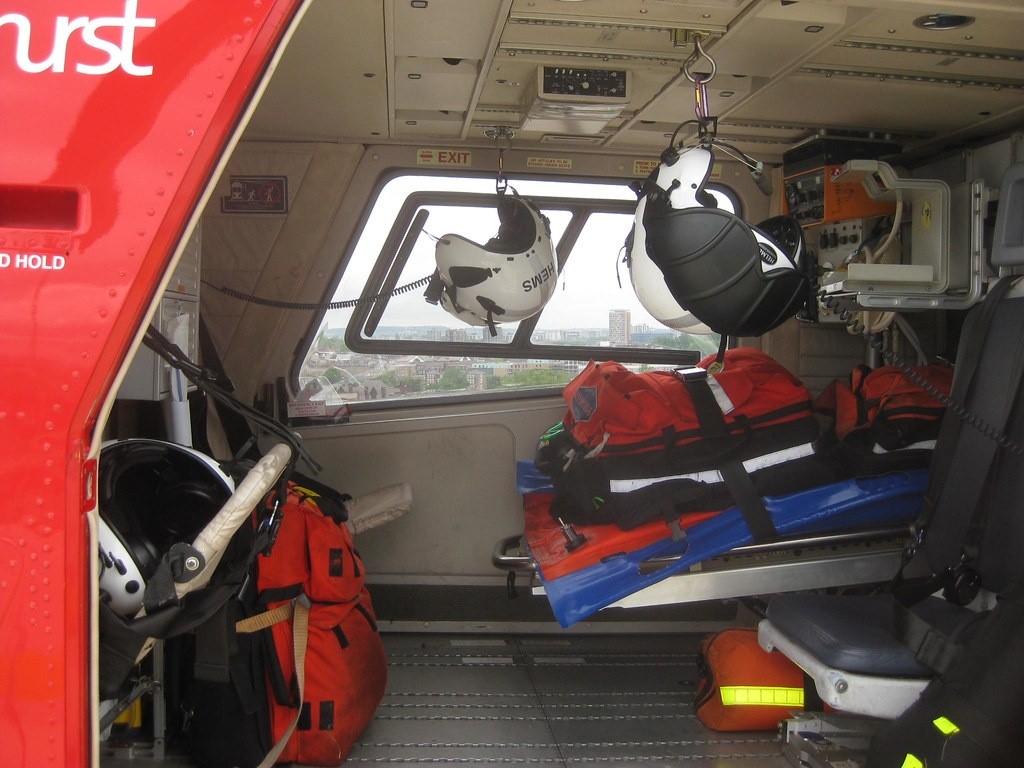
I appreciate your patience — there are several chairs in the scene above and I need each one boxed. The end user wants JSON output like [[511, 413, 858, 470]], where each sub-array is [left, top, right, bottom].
[[757, 294, 1024, 720]]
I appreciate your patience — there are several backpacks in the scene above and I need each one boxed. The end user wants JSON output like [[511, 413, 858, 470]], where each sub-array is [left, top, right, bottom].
[[867, 582, 1024, 768], [192, 460, 386, 767], [535, 348, 950, 529]]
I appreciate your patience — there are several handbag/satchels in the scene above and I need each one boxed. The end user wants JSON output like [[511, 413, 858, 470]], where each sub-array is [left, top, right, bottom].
[[694, 630, 836, 731]]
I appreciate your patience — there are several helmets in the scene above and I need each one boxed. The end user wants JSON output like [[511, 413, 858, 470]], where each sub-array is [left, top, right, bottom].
[[622, 145, 810, 338], [436, 197, 558, 325], [90, 439, 258, 638]]
